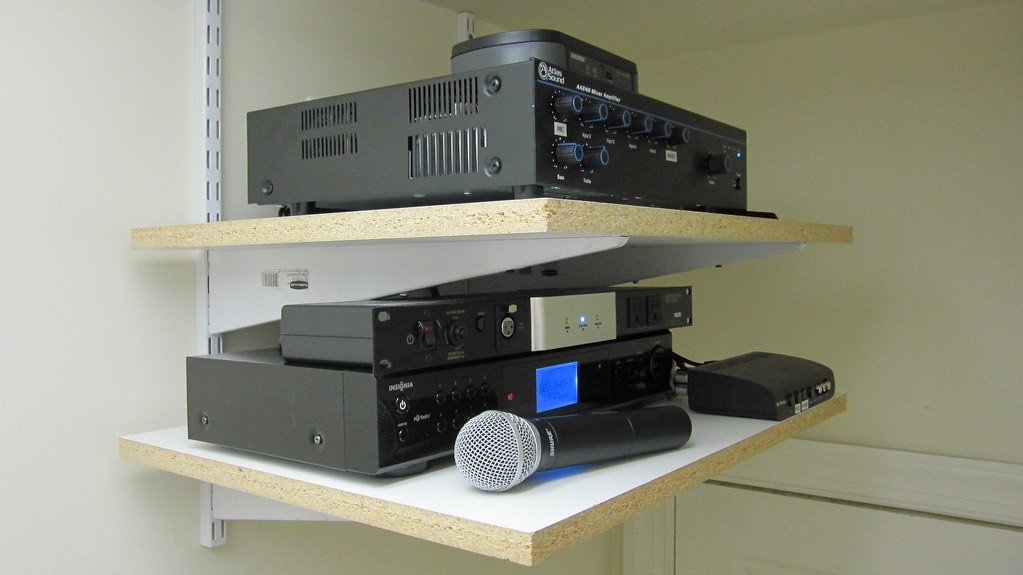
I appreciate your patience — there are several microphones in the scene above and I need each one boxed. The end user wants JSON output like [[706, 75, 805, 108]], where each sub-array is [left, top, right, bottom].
[[453, 405, 692, 493]]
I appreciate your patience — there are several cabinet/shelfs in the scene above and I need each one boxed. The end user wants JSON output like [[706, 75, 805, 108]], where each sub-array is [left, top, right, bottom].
[[118, 196, 853, 567]]
